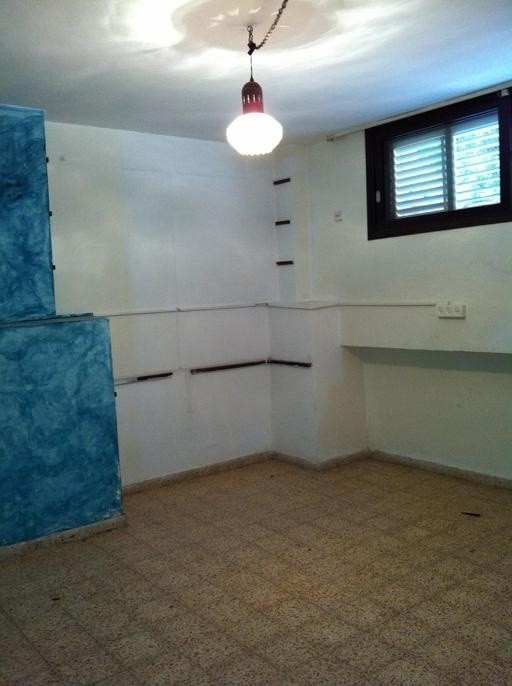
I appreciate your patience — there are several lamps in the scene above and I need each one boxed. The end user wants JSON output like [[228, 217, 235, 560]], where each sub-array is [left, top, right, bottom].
[[226, 1, 288, 155]]
